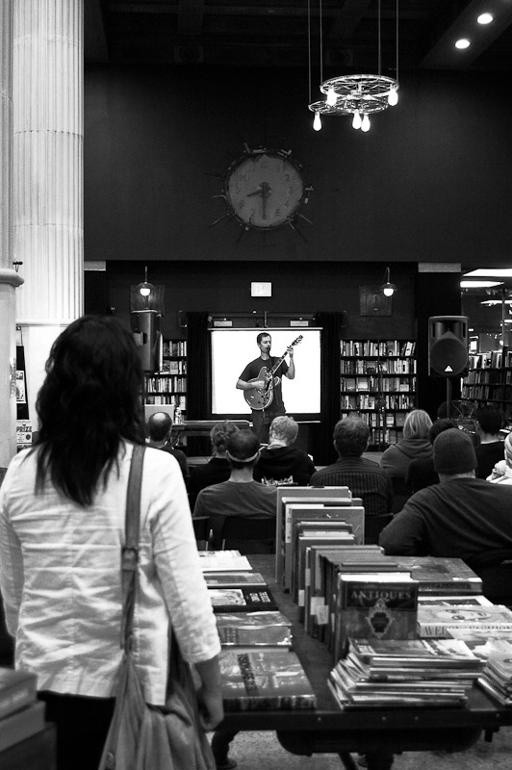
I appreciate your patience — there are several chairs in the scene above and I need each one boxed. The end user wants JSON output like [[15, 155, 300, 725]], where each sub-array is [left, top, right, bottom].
[[365, 457, 439, 546], [184, 463, 276, 551]]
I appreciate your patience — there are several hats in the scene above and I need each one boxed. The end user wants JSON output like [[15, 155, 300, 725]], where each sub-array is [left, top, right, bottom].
[[432, 427, 479, 474]]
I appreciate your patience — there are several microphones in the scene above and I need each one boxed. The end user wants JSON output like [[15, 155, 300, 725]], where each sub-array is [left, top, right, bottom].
[[267, 347, 272, 360]]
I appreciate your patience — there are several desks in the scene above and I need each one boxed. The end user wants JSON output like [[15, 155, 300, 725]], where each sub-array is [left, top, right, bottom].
[[192, 555, 512, 770], [167, 419, 250, 459]]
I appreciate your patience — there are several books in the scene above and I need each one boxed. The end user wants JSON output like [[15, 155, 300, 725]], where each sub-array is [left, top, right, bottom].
[[138, 339, 187, 423], [199, 551, 318, 712], [276, 484, 512, 709], [341, 339, 419, 445], [459, 347, 512, 429]]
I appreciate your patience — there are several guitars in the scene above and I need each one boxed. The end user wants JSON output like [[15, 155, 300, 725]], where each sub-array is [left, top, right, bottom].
[[244, 335, 303, 410]]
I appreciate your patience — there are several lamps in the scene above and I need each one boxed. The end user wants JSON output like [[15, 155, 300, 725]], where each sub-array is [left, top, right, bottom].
[[380, 264, 396, 299], [138, 266, 154, 300], [307, 1, 398, 133]]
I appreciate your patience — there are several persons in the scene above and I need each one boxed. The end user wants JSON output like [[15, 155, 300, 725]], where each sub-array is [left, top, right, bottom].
[[383, 429, 511, 606], [249, 415, 320, 488], [379, 408, 435, 476], [305, 416, 396, 517], [192, 422, 243, 500], [145, 413, 188, 477], [192, 428, 278, 546], [232, 331, 305, 435], [2, 314, 226, 768], [408, 418, 459, 488]]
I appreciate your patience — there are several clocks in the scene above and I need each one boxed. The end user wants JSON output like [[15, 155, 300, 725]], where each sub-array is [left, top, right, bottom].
[[208, 139, 317, 250]]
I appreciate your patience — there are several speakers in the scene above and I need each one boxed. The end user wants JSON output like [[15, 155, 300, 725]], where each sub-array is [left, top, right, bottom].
[[428, 315, 469, 379], [131, 310, 160, 372]]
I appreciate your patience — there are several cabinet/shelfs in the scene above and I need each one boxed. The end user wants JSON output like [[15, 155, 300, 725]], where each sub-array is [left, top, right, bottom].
[[339, 337, 421, 455], [132, 336, 189, 449], [461, 351, 512, 434]]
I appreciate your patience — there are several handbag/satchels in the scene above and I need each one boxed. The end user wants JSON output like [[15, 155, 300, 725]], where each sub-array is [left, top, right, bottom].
[[98, 652, 218, 770]]
